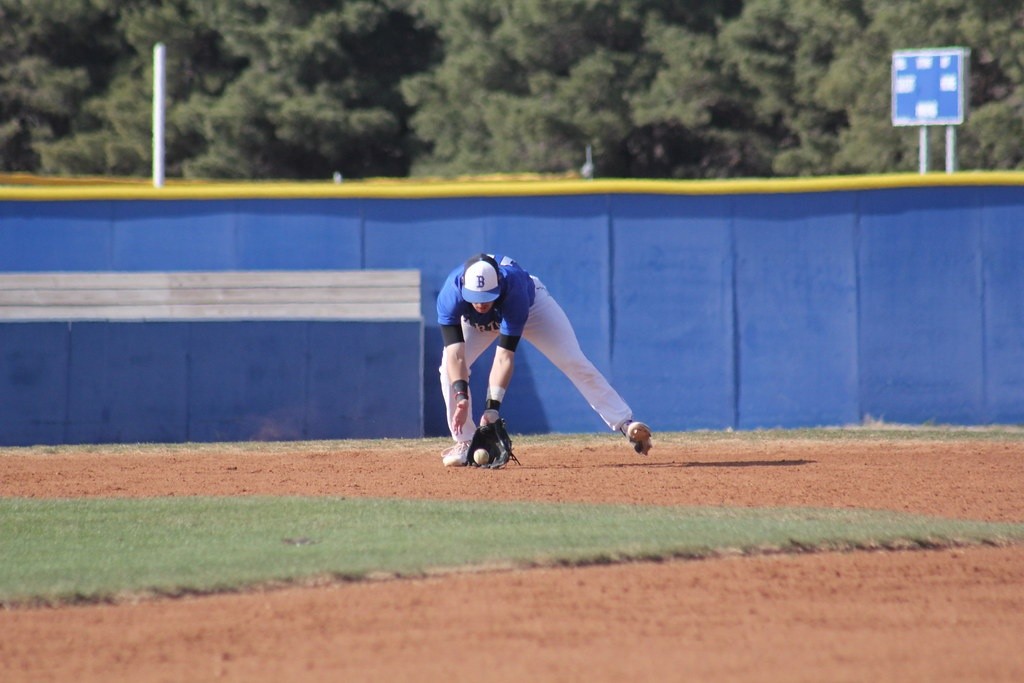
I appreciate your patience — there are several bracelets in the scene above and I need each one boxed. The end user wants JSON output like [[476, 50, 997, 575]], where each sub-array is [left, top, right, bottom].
[[452, 379, 469, 404], [483, 386, 506, 415]]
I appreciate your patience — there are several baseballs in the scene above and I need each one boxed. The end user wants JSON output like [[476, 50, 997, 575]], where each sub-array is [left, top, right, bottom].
[[473, 449, 489, 465]]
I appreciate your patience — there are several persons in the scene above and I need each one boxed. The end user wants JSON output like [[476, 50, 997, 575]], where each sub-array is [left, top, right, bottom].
[[436, 253, 652, 467]]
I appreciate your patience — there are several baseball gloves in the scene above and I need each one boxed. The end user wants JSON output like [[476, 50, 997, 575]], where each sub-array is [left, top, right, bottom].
[[465, 418, 512, 469]]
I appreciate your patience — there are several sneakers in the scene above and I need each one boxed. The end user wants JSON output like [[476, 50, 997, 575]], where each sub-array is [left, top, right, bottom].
[[441, 440, 472, 466], [626, 420, 653, 455]]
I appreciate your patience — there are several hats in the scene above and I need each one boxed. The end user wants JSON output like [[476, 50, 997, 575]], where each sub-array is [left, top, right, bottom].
[[462, 261, 501, 303]]
